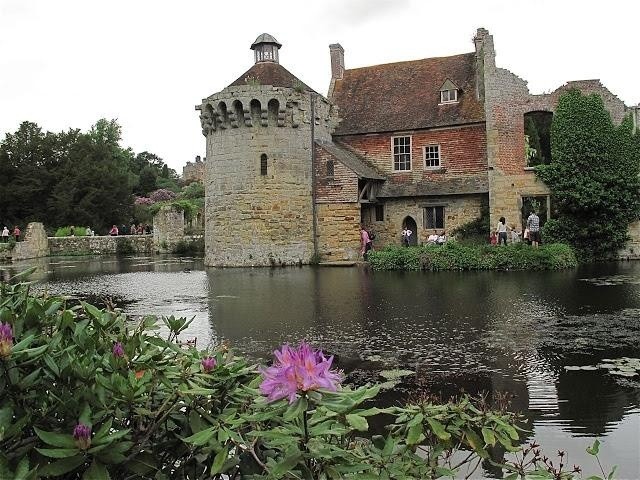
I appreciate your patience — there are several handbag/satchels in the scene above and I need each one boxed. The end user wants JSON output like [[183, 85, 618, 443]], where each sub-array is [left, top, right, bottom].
[[524, 226, 530, 239]]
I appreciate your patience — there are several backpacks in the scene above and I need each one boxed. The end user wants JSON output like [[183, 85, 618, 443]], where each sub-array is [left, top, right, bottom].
[[365, 230, 375, 240]]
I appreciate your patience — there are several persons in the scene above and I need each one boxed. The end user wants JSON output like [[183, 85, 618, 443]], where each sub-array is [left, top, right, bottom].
[[424, 229, 447, 247], [85, 226, 92, 236], [361, 224, 369, 258], [108, 223, 153, 236], [489, 217, 522, 244], [1, 227, 11, 243], [14, 226, 21, 242], [400, 227, 412, 248], [70, 225, 75, 237], [527, 210, 540, 250]]
